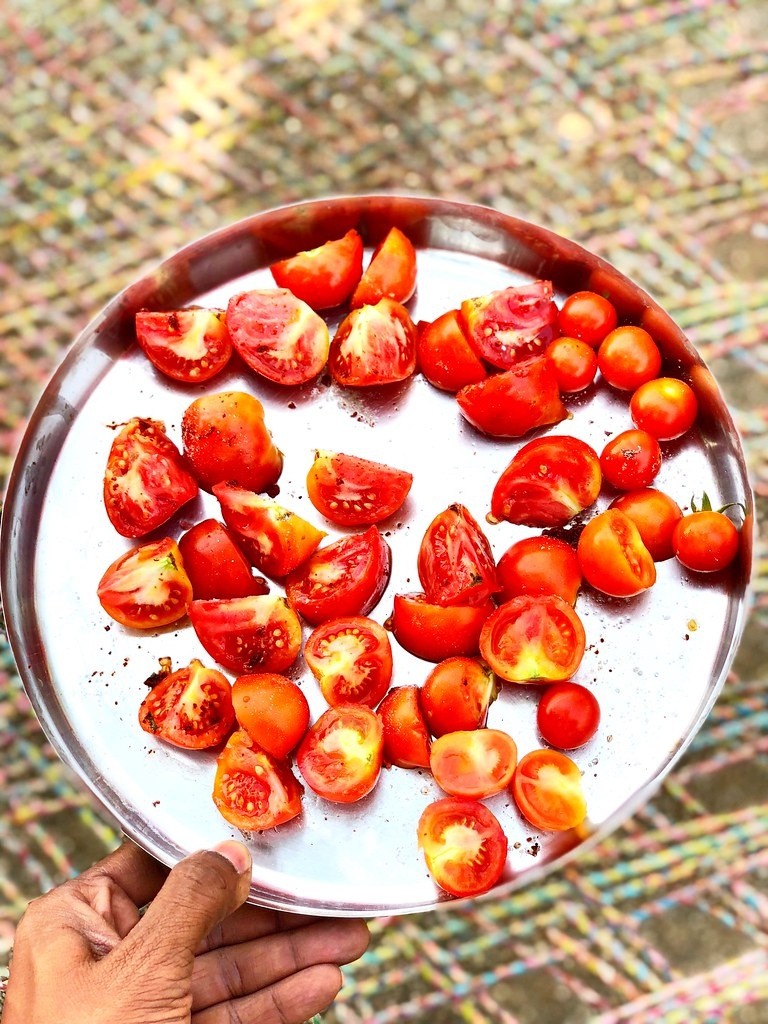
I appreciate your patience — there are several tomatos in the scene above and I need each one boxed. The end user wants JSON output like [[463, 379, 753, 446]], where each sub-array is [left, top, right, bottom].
[[98, 228, 746, 897]]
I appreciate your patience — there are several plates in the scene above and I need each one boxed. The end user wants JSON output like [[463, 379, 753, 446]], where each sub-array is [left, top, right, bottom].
[[0, 194, 757, 918]]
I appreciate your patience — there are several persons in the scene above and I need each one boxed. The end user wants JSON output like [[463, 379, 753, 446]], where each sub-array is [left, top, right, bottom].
[[0, 841, 370, 1024]]
